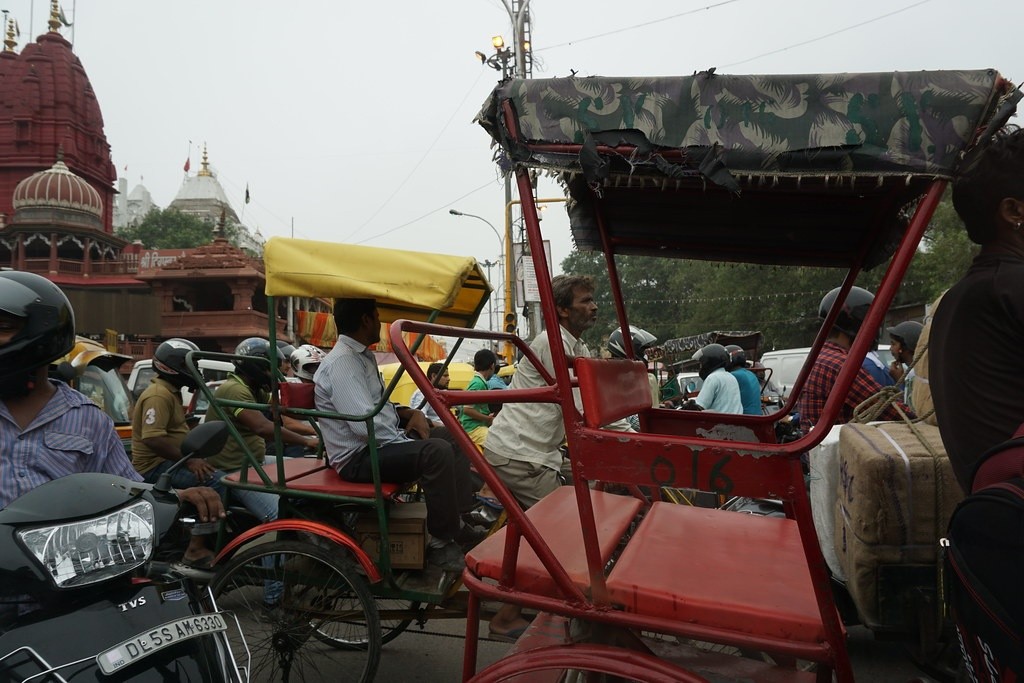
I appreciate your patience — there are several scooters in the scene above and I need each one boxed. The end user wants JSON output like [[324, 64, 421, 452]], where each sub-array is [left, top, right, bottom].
[[0, 420, 254, 683]]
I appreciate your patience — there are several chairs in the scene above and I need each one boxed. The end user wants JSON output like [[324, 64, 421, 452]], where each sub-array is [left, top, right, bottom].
[[282, 383, 401, 497], [580, 351, 843, 644]]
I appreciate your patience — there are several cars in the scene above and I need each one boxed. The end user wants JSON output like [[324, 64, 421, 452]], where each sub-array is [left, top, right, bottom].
[[186, 380, 228, 424]]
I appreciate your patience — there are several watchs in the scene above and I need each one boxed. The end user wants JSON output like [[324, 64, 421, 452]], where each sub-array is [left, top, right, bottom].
[[788, 416, 793, 422]]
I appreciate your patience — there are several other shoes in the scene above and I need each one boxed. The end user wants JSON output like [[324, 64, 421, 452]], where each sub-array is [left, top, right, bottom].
[[426, 539, 467, 572], [453, 522, 489, 549]]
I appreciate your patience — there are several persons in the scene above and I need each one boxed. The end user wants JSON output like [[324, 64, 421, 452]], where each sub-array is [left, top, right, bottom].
[[204, 338, 319, 624], [724, 345, 763, 415], [0, 271, 226, 523], [607, 325, 657, 432], [928, 127, 1024, 494], [276, 339, 327, 458], [460, 349, 506, 449], [410, 362, 451, 426], [483, 274, 638, 644], [313, 298, 485, 570], [691, 344, 743, 414], [779, 286, 924, 439], [131, 338, 226, 569]]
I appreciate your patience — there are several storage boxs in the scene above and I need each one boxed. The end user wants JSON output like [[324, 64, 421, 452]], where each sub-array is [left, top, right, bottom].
[[362, 504, 426, 568]]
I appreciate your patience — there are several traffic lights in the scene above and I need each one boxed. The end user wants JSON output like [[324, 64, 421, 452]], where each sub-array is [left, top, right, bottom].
[[503, 312, 516, 346]]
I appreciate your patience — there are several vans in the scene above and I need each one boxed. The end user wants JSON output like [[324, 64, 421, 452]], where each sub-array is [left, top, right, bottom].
[[127, 359, 235, 418], [760, 343, 902, 411]]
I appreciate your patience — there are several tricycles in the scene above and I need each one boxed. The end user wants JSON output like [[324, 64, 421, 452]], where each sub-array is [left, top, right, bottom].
[[381, 66, 1024, 683], [130, 235, 794, 683], [641, 329, 774, 415]]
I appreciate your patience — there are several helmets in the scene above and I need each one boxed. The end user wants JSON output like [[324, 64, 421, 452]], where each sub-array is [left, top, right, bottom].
[[699, 344, 730, 381], [723, 345, 746, 371], [819, 286, 883, 350], [750, 363, 765, 381], [606, 325, 649, 368], [233, 338, 283, 392], [151, 338, 205, 386], [0, 271, 76, 382], [272, 340, 295, 377], [886, 320, 925, 353], [289, 344, 327, 381]]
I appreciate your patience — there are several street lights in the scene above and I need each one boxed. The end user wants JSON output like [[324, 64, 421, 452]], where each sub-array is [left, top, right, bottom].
[[478, 258, 500, 353], [449, 208, 508, 301], [473, 35, 529, 367]]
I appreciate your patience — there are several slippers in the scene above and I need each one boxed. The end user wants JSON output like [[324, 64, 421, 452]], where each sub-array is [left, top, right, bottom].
[[488, 625, 529, 643], [181, 553, 223, 571]]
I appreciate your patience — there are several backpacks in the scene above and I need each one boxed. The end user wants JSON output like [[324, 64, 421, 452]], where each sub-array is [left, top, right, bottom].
[[942, 423, 1024, 682]]
